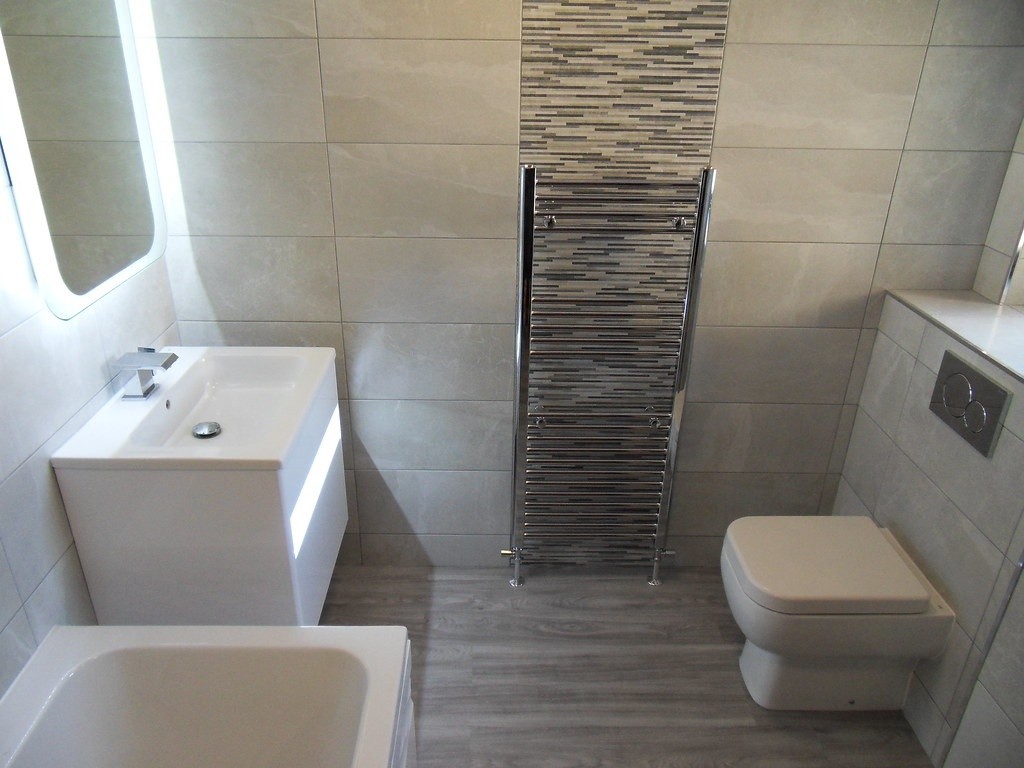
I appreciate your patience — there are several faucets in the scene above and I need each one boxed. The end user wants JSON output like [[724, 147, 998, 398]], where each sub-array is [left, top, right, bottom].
[[112, 351, 179, 399]]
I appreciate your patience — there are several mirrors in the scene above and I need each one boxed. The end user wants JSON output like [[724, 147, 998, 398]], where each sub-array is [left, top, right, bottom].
[[0, 0, 167, 319]]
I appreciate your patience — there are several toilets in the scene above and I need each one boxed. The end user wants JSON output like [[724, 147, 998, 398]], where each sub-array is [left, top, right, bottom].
[[715, 514, 957, 713]]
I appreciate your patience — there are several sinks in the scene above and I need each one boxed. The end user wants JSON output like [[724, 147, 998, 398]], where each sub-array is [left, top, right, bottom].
[[122, 352, 304, 452]]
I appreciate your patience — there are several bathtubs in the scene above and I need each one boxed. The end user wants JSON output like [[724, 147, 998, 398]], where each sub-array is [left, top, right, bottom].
[[1, 626, 420, 768]]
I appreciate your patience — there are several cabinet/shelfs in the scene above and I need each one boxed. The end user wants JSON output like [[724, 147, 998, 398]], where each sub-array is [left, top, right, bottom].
[[53, 357, 350, 624], [387, 640, 415, 767]]
